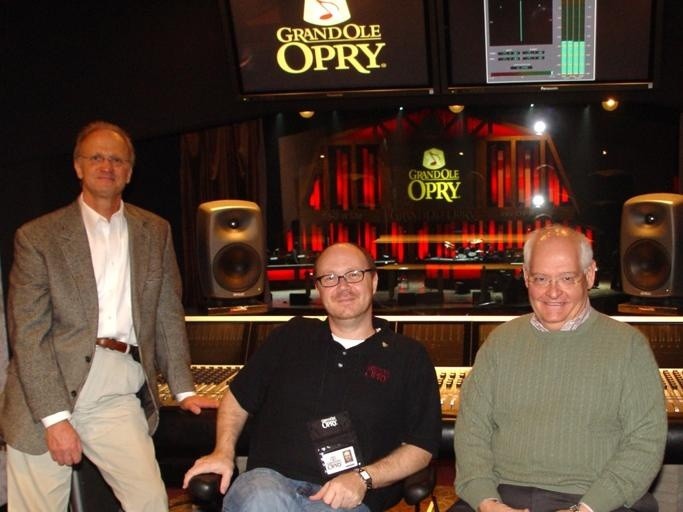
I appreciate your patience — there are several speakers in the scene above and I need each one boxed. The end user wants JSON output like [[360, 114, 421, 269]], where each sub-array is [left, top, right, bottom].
[[196, 200, 268, 316], [617, 193, 683, 315]]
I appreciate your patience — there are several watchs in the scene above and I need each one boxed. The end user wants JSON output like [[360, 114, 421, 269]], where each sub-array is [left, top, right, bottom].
[[352, 466, 372, 490]]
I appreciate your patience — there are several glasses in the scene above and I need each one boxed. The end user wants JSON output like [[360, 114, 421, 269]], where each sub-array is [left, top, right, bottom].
[[316, 268, 373, 288], [526, 270, 587, 290], [77, 153, 131, 168]]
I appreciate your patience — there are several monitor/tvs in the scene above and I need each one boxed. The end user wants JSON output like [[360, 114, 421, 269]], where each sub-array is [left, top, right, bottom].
[[220, 0, 441, 104], [435, 0, 657, 96]]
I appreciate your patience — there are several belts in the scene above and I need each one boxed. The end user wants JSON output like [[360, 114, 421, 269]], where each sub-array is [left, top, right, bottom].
[[94, 337, 139, 358]]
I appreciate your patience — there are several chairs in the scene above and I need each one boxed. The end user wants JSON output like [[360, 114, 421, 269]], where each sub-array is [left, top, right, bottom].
[[184, 454, 439, 512], [441, 425, 456, 447], [68, 454, 124, 512]]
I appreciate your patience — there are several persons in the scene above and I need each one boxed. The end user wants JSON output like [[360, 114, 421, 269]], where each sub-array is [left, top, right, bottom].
[[180, 241, 444, 512], [342, 450, 351, 462], [444, 221, 668, 511], [0, 117, 220, 512]]
